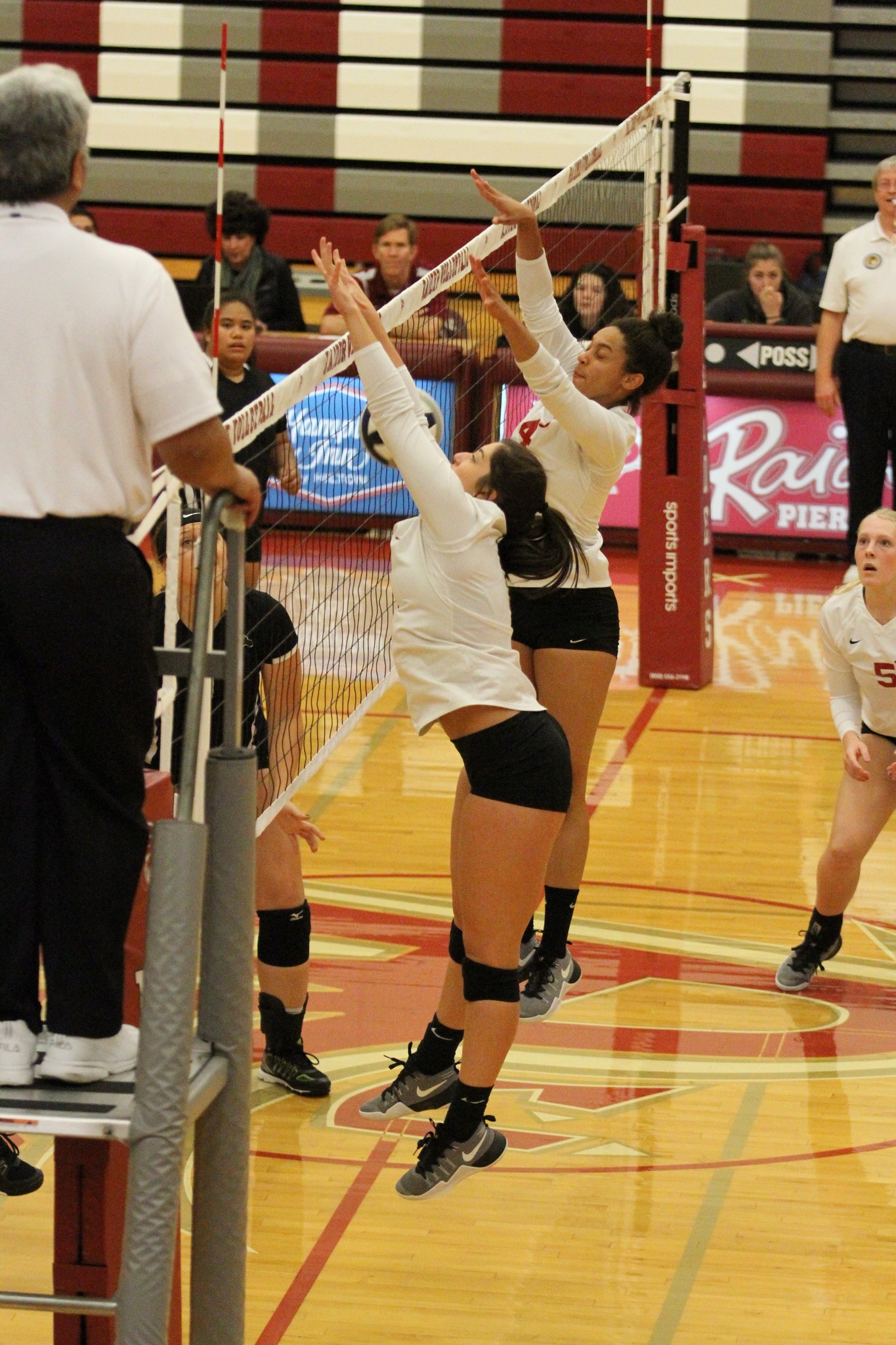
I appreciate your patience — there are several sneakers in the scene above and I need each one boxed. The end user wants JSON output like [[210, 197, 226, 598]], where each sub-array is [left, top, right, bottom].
[[37, 1024, 139, 1084], [359, 1041, 462, 1120], [519, 941, 582, 1024], [257, 1036, 331, 1095], [775, 930, 842, 991], [517, 929, 544, 984], [0, 1132, 44, 1196], [0, 1020, 38, 1085], [396, 1115, 508, 1201]]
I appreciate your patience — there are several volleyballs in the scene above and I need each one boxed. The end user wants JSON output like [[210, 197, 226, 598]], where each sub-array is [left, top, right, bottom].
[[358, 387, 443, 468]]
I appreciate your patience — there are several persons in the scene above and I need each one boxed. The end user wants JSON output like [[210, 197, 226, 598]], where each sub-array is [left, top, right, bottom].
[[69, 147, 895, 1202], [0, 59, 262, 1087]]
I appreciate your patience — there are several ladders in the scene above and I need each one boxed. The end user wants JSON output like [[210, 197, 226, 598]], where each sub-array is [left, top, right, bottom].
[[0, 489, 257, 1345]]
